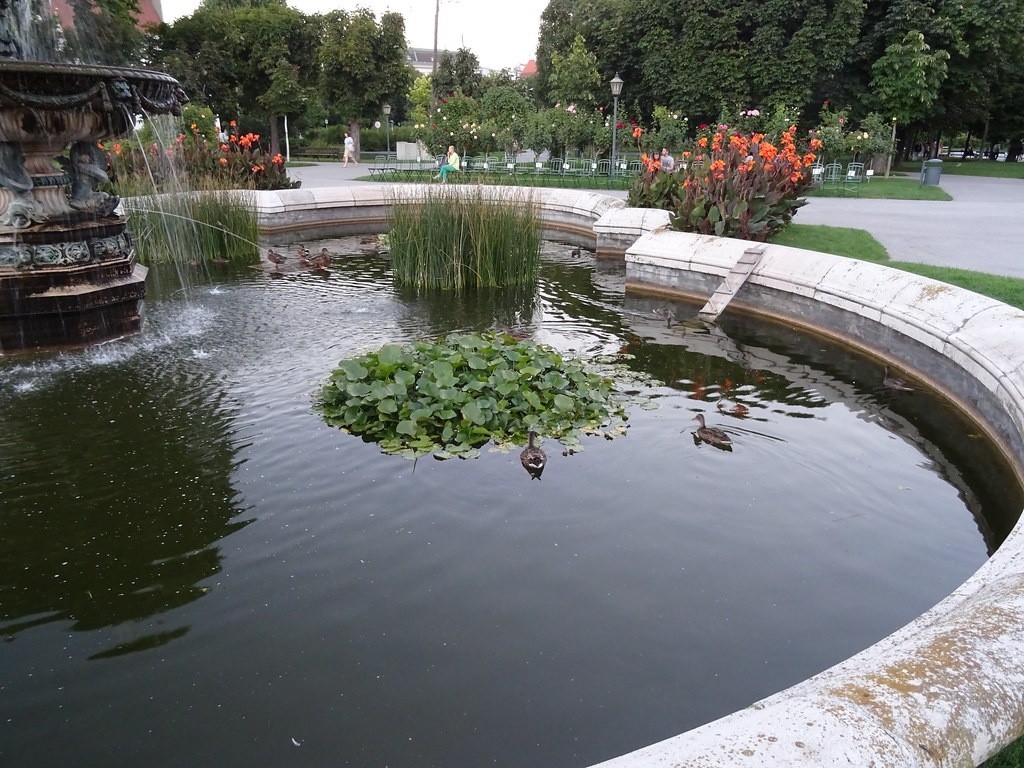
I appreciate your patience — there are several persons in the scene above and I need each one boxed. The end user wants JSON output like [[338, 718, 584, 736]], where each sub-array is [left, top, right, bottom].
[[912, 141, 944, 159], [513, 142, 523, 156], [641, 149, 661, 173], [432, 146, 459, 185], [969, 138, 1024, 161], [659, 147, 674, 174], [219, 127, 230, 144], [343, 132, 359, 167]]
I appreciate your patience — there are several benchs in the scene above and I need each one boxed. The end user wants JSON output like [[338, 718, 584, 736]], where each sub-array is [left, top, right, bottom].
[[293, 147, 318, 161], [983, 151, 990, 157], [961, 149, 978, 158], [313, 148, 341, 162]]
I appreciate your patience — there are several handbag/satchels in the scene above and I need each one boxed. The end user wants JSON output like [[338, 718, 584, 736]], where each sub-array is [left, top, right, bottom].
[[349, 144, 355, 151]]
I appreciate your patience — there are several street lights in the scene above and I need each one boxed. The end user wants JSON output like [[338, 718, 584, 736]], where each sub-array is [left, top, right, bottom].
[[382, 101, 392, 160], [609, 72, 624, 183]]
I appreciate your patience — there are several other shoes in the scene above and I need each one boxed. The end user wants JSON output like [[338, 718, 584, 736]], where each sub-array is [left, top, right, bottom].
[[342, 165, 347, 168], [433, 174, 441, 181], [356, 163, 359, 167], [440, 180, 448, 185]]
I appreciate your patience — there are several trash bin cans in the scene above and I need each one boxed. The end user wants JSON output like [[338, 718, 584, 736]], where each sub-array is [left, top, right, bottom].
[[920, 159, 943, 186]]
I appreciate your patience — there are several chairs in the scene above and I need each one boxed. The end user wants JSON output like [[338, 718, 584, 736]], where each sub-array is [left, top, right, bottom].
[[367, 155, 386, 181], [625, 159, 643, 189], [594, 159, 611, 189], [512, 157, 530, 186], [530, 157, 548, 187], [429, 154, 447, 184], [822, 164, 842, 197], [609, 159, 628, 190], [691, 161, 704, 169], [576, 159, 594, 189], [842, 162, 864, 198], [458, 156, 473, 183], [545, 158, 564, 187], [496, 156, 515, 185], [479, 156, 499, 185], [674, 160, 689, 170], [810, 163, 823, 196], [561, 158, 578, 188], [382, 154, 432, 182], [469, 156, 485, 185]]
